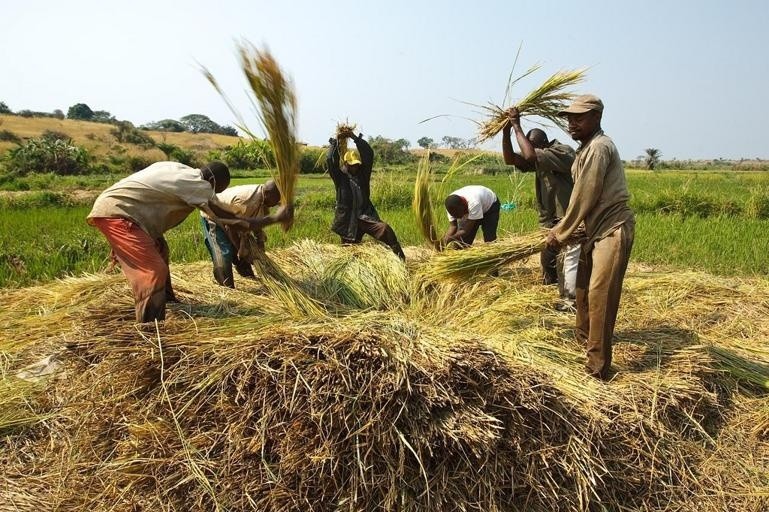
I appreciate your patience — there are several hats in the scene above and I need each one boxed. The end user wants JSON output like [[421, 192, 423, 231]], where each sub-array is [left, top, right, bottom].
[[345, 150, 362, 166], [558, 94, 603, 117]]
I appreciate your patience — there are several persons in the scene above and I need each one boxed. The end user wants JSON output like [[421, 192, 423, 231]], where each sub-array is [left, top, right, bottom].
[[541, 90, 636, 383], [501, 104, 576, 285], [195, 176, 290, 287], [89, 158, 232, 326], [433, 184, 501, 282], [326, 123, 406, 262]]
